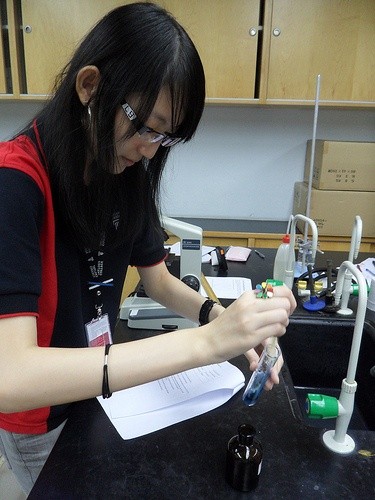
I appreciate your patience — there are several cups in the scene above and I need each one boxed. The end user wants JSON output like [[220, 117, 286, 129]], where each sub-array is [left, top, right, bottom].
[[366, 276, 375, 312], [297, 247, 314, 275]]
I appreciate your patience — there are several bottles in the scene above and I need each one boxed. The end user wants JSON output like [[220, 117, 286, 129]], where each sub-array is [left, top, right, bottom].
[[273, 234, 296, 290]]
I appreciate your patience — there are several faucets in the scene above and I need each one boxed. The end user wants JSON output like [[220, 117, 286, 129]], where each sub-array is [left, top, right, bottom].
[[285, 214, 318, 291], [321, 260, 369, 456], [334, 214, 364, 317]]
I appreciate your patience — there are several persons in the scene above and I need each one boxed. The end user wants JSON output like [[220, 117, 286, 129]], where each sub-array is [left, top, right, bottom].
[[0, 0, 297, 499]]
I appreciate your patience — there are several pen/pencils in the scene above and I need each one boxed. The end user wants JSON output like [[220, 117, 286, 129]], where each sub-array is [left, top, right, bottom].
[[366, 269, 375, 276], [255, 250, 264, 257]]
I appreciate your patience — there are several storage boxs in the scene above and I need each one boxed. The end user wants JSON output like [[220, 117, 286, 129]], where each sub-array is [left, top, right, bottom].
[[292, 139, 375, 238]]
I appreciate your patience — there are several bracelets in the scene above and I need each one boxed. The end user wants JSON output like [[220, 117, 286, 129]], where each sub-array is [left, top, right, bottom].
[[198, 298, 218, 326], [102, 343, 112, 399]]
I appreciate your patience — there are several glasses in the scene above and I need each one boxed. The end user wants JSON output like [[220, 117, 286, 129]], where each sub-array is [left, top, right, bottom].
[[119, 99, 182, 148]]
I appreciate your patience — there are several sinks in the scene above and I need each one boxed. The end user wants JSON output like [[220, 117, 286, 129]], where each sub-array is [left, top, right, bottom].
[[277, 313, 375, 432]]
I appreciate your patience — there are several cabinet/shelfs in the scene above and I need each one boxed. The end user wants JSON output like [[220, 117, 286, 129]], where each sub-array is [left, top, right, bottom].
[[0, 0, 375, 108]]
